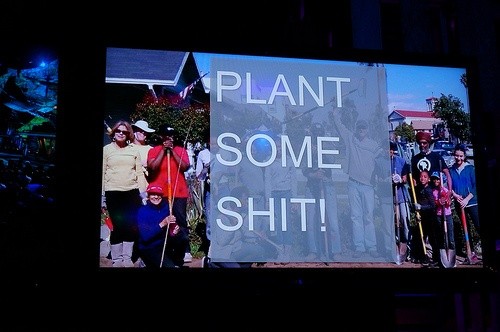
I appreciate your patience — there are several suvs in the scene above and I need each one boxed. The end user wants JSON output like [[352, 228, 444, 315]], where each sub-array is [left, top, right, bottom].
[[0, 131, 58, 183]]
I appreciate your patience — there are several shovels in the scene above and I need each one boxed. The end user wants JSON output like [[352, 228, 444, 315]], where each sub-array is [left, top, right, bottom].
[[440, 173, 456, 268], [392, 171, 407, 265]]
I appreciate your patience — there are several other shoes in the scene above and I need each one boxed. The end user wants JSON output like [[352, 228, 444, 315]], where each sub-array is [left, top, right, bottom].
[[184, 253, 193, 263], [331, 253, 342, 261], [352, 250, 362, 257], [305, 253, 317, 262], [370, 251, 379, 257]]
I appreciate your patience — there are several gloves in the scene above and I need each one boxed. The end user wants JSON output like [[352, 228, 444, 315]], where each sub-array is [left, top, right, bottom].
[[391, 173, 402, 184], [413, 202, 421, 210]]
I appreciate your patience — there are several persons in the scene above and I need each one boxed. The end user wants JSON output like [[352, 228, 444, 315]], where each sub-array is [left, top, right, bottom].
[[195, 96, 483, 268], [101, 120, 193, 268]]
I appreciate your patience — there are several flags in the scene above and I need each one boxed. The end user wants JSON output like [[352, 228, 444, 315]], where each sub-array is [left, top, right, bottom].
[[176, 72, 209, 105]]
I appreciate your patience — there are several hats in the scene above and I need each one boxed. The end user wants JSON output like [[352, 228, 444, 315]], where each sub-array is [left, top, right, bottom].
[[158, 123, 178, 135], [147, 184, 163, 195], [415, 132, 431, 146], [131, 121, 155, 133], [430, 171, 447, 183], [356, 119, 368, 129]]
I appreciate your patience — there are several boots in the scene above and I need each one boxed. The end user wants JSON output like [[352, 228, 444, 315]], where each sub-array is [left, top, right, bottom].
[[123, 241, 134, 267], [110, 242, 123, 267]]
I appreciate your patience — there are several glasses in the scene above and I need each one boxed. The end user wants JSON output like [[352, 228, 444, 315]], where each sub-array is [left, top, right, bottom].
[[115, 128, 128, 135]]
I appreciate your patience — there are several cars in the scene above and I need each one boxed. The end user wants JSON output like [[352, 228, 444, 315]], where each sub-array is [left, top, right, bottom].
[[428, 140, 474, 165]]
